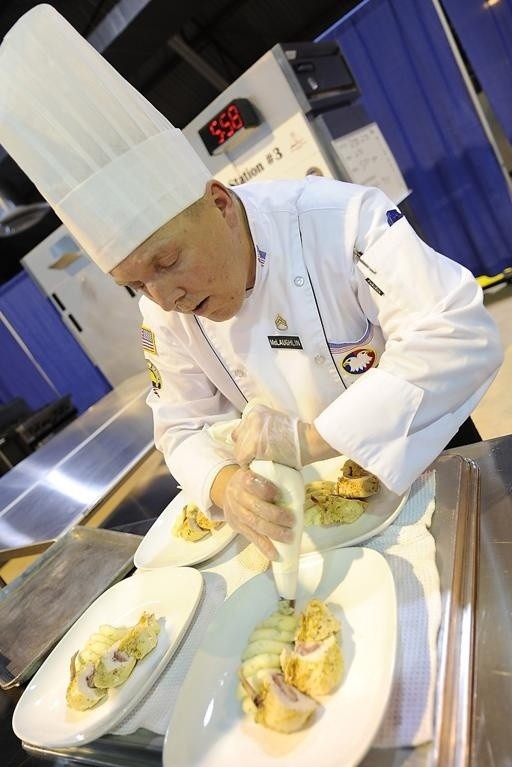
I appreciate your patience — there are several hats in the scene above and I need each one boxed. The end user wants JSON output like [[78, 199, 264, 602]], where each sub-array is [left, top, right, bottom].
[[0, 3, 215, 277]]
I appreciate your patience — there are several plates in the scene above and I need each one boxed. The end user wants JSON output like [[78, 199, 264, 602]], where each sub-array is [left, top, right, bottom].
[[11, 567, 203, 748], [162, 546, 398, 767], [134, 488, 242, 569], [298, 454, 413, 558]]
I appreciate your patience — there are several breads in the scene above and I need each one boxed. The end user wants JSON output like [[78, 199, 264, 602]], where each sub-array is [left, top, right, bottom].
[[303, 457, 379, 525], [170, 503, 217, 542], [239, 600, 345, 736], [65, 610, 157, 713]]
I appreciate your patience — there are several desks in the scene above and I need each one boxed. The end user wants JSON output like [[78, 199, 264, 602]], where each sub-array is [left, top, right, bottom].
[[4, 436, 511, 765], [4, 370, 179, 583]]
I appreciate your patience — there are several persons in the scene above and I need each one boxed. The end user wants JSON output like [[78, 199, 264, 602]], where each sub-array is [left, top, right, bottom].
[[104, 176, 509, 570]]
